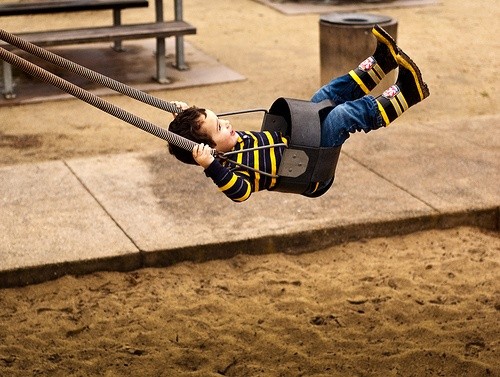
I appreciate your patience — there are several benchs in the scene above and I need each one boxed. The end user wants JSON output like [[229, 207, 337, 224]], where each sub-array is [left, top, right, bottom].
[[0, 19, 197, 101], [0, 0, 149, 52]]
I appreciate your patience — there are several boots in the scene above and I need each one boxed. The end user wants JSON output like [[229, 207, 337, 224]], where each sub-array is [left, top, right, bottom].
[[375, 50, 430, 127], [347, 23, 404, 95]]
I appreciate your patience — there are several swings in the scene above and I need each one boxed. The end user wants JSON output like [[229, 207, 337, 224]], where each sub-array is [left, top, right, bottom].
[[1, 28, 343, 195]]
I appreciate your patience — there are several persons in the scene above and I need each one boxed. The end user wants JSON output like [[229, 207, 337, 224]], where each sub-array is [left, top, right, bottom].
[[168, 23, 430, 203]]
